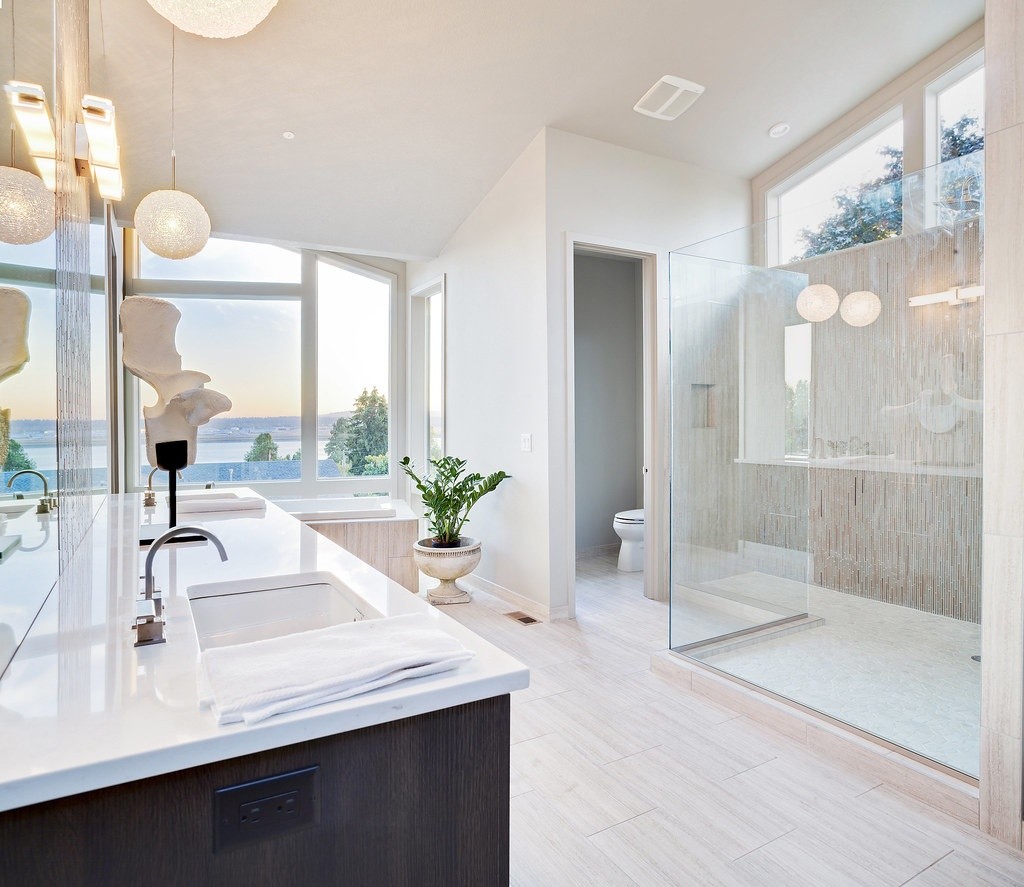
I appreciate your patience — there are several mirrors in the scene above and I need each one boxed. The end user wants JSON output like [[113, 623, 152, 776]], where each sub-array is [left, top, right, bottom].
[[1, 1, 59, 676]]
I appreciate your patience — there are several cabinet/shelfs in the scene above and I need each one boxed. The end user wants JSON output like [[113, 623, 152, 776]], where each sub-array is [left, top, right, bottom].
[[306, 522, 416, 590], [0, 691, 509, 887]]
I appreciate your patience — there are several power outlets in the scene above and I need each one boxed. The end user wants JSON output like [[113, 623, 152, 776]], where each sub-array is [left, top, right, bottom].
[[213, 766, 320, 854]]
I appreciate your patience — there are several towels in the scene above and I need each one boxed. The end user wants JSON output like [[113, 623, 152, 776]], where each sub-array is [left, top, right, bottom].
[[193, 612, 480, 725], [176, 497, 266, 513]]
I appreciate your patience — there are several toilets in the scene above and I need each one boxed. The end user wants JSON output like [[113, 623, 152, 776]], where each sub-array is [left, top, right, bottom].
[[612, 508, 646, 572]]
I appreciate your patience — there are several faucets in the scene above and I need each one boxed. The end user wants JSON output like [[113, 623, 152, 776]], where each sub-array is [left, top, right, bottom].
[[148, 463, 183, 491], [144, 523, 230, 600], [7, 469, 49, 496]]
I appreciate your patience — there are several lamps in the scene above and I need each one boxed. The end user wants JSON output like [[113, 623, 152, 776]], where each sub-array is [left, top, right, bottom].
[[3, 81, 64, 191], [132, 158, 211, 261], [81, 95, 129, 200], [1, 125, 56, 245]]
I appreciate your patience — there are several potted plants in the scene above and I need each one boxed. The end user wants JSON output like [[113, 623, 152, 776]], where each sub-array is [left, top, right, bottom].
[[397, 454, 511, 606]]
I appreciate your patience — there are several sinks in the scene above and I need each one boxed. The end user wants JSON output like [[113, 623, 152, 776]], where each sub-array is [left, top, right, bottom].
[[165, 492, 241, 507], [186, 570, 388, 653]]
